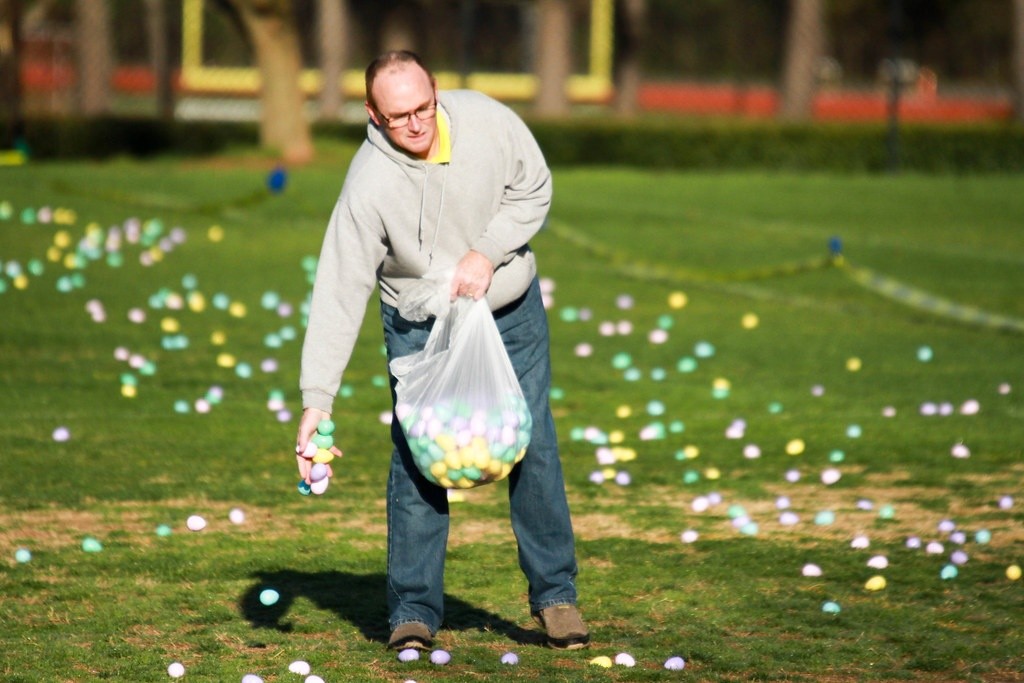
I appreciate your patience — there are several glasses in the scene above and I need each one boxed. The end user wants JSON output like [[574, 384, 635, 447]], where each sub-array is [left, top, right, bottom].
[[368, 97, 437, 128]]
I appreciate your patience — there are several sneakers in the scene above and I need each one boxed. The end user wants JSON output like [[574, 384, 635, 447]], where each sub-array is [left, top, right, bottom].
[[532, 604, 590, 650], [388, 622, 432, 651]]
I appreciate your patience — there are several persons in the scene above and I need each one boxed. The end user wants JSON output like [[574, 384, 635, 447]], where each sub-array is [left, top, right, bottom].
[[296, 50, 592, 651]]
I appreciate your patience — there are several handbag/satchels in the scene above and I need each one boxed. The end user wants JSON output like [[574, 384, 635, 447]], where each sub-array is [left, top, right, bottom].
[[389, 261, 532, 488]]
